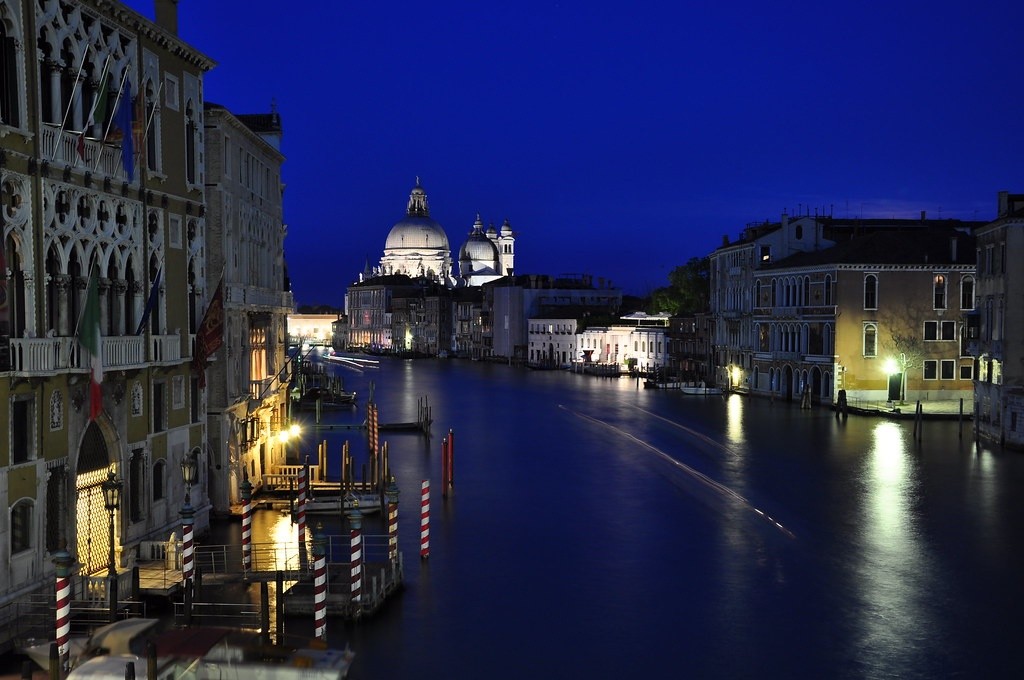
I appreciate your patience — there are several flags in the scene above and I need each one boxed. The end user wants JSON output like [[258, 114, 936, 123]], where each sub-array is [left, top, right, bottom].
[[75, 64, 110, 162], [77, 271, 103, 420], [114, 78, 134, 184], [110, 86, 145, 164], [136, 270, 161, 335], [193, 281, 223, 390]]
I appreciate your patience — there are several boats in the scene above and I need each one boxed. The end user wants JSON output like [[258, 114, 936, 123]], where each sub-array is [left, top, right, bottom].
[[679, 388, 724, 394], [304, 494, 394, 515], [377, 419, 434, 430]]
